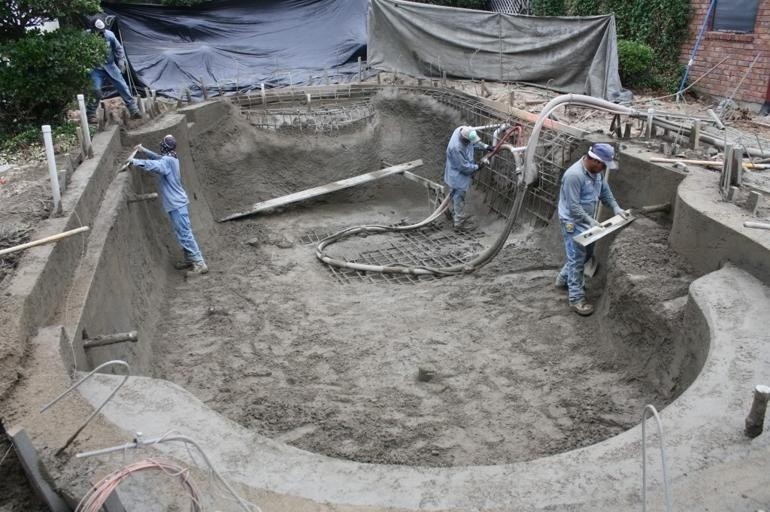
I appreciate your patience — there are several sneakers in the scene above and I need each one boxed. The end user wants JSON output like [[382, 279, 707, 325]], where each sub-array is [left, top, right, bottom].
[[186, 262, 208, 277], [454, 223, 477, 231], [175, 260, 192, 270], [555, 279, 567, 286], [132, 111, 143, 119], [570, 302, 594, 315], [88, 117, 98, 124]]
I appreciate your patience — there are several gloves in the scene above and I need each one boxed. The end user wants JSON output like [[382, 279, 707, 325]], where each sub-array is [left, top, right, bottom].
[[613, 206, 628, 220], [119, 64, 125, 73], [134, 145, 145, 152], [125, 157, 134, 164], [584, 217, 603, 228], [486, 146, 496, 151], [478, 162, 486, 170]]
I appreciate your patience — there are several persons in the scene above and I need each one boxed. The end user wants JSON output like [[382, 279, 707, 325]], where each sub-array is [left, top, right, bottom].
[[85, 19, 143, 124], [125, 134, 208, 278], [443, 125, 497, 230], [554, 143, 630, 316]]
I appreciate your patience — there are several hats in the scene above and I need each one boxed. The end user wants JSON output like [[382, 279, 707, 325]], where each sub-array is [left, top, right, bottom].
[[588, 143, 616, 169], [164, 134, 177, 149], [92, 18, 106, 33], [461, 126, 481, 144]]
